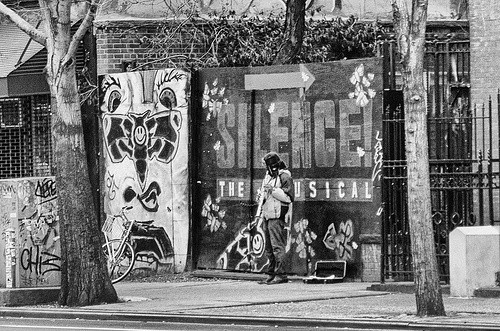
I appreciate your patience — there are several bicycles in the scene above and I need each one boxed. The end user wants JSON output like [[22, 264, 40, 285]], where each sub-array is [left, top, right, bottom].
[[103, 204, 153, 289]]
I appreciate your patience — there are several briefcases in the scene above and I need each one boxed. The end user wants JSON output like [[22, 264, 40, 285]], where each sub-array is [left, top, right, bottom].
[[303, 259, 348, 284]]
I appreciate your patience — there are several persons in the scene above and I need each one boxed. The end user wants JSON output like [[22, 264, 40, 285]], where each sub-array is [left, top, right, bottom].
[[255, 152, 296, 284]]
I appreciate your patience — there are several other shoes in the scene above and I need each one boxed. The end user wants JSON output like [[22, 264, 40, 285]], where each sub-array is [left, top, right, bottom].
[[257, 275, 275, 284], [265, 273, 288, 285]]
